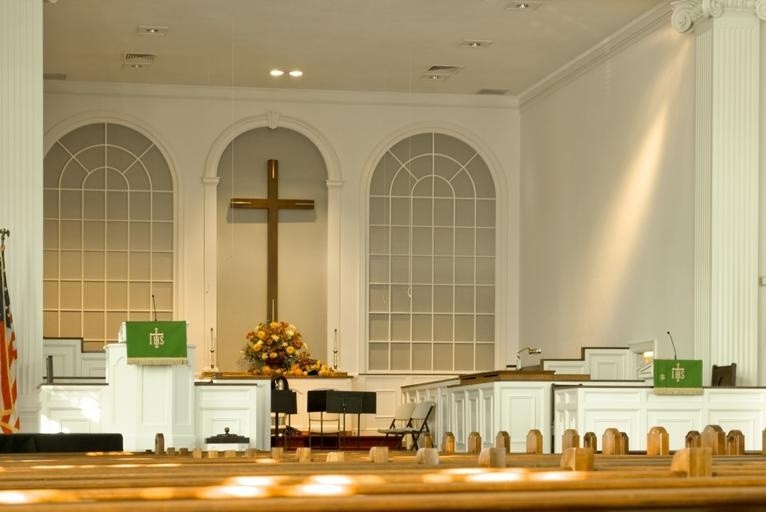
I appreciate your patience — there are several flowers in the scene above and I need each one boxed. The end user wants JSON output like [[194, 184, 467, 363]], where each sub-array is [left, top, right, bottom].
[[239, 322, 334, 376]]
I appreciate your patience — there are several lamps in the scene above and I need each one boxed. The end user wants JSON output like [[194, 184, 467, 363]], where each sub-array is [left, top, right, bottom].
[[515, 346, 541, 371]]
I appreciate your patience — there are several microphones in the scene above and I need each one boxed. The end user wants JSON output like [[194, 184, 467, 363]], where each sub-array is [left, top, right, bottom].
[[151, 294, 157, 321], [667, 332, 677, 360]]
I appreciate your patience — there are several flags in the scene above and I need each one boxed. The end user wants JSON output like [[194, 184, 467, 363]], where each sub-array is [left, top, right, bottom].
[[0, 226, 22, 436]]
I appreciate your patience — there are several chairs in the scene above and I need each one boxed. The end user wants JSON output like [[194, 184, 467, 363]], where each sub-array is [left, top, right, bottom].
[[377, 402, 434, 450]]
